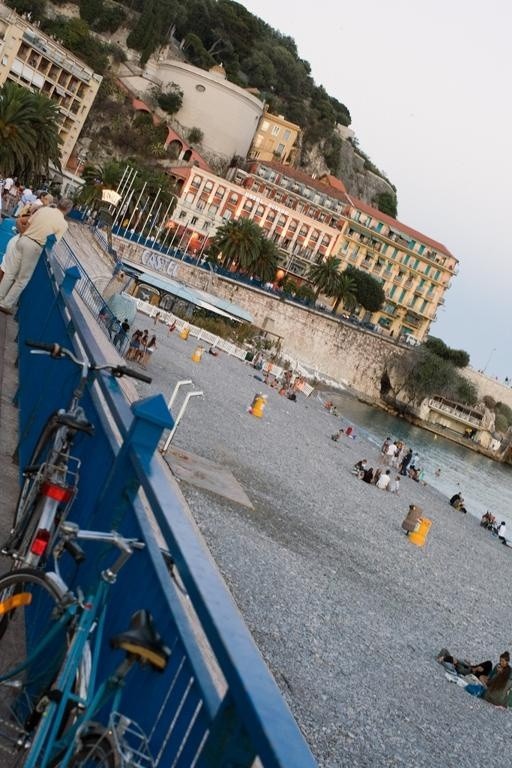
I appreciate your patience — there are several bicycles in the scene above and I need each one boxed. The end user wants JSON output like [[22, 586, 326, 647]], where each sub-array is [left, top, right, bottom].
[[0, 511, 175, 762], [0, 328, 157, 631]]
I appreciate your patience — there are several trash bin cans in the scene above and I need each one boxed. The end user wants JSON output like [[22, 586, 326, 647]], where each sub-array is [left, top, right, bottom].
[[249, 392, 267, 417], [191, 345, 205, 362], [402, 504, 432, 547], [179, 327, 191, 340], [0, 217, 20, 264]]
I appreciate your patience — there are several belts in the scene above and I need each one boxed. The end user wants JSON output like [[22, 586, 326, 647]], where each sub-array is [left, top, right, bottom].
[[26, 235, 41, 246]]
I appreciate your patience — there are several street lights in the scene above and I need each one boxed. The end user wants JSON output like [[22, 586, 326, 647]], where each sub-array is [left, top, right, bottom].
[[481, 346, 497, 375], [58, 150, 87, 202]]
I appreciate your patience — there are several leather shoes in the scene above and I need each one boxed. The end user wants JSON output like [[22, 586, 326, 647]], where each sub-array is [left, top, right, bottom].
[[0, 306, 16, 315]]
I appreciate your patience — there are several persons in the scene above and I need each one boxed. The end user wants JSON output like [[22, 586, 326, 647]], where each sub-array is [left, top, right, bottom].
[[437, 647, 494, 679], [254, 352, 338, 417], [493, 520, 506, 537], [93, 304, 179, 370], [328, 424, 443, 495], [0, 198, 74, 315], [1, 172, 55, 286], [478, 651, 510, 690], [449, 491, 462, 507], [482, 664, 511, 707], [209, 343, 219, 356], [451, 497, 465, 512]]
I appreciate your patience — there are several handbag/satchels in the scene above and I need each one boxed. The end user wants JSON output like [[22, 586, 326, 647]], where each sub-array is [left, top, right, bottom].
[[443, 660, 458, 675]]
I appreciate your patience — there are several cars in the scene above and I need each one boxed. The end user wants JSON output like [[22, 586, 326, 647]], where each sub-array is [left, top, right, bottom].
[[265, 278, 424, 351]]
